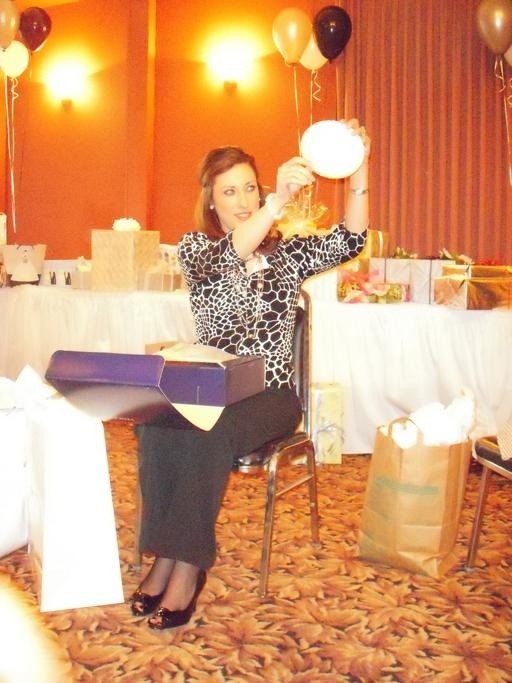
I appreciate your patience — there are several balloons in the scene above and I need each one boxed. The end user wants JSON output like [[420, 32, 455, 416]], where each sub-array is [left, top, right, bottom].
[[271, 5, 311, 63], [313, 4, 352, 60], [17, 5, 51, 51], [299, 23, 329, 71], [501, 44, 511, 67], [0, 0, 17, 47], [1, 41, 31, 77], [476, 0, 511, 58]]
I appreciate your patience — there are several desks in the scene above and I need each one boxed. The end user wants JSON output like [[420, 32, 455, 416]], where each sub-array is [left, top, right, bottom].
[[284, 253, 512, 468], [1, 253, 196, 387]]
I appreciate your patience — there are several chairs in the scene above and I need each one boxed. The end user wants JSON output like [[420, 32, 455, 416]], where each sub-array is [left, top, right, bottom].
[[128, 285, 322, 602]]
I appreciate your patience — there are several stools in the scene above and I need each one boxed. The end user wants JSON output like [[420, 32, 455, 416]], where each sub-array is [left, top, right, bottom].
[[464, 432, 511, 569]]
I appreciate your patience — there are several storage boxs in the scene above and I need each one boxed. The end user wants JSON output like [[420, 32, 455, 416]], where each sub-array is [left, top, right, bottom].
[[42, 331, 268, 435], [140, 269, 184, 293], [86, 224, 163, 292], [333, 228, 512, 310]]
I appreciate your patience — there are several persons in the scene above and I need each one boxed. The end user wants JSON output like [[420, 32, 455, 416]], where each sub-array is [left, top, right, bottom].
[[127, 119, 373, 632]]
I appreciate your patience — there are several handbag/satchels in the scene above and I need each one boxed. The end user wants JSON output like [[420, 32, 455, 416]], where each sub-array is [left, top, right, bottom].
[[0, 389, 51, 562], [24, 393, 125, 615], [354, 408, 477, 582]]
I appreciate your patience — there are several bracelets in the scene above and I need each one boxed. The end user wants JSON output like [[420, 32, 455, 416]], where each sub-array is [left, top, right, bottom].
[[264, 192, 286, 220], [348, 186, 369, 195]]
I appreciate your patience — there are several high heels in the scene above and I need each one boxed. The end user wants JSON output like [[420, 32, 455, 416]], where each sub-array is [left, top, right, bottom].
[[129, 584, 167, 617], [148, 566, 208, 631]]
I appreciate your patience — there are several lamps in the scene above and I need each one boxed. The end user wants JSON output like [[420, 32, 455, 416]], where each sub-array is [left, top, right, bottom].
[[61, 97, 73, 112], [224, 80, 237, 94]]
